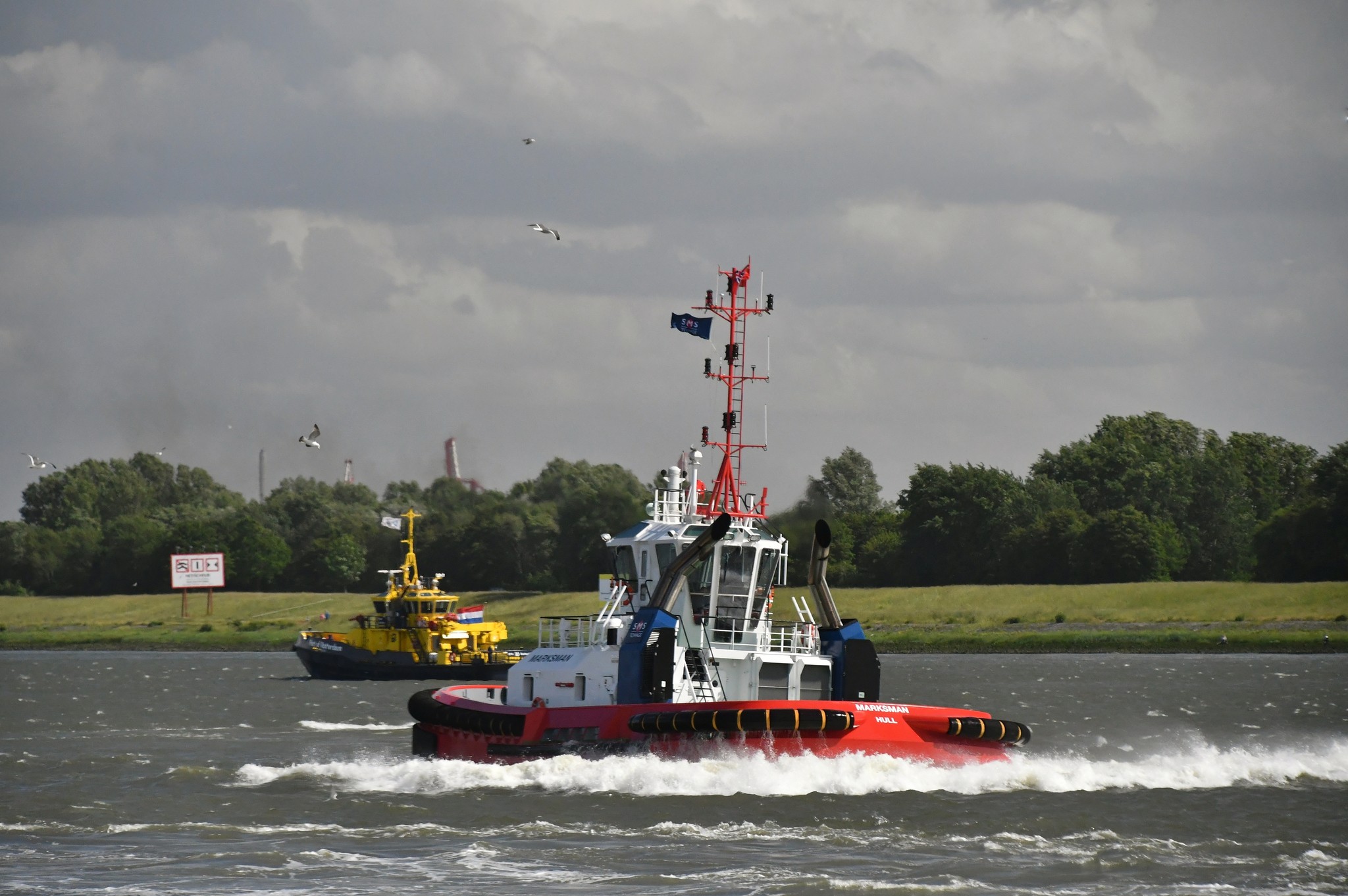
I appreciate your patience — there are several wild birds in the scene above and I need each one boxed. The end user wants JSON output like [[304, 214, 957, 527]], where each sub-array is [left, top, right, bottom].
[[526, 222, 561, 242], [521, 137, 536, 145], [19, 451, 57, 471], [150, 446, 167, 457], [297, 423, 322, 451]]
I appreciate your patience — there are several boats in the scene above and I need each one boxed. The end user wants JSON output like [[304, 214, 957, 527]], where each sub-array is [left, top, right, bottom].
[[409, 258, 1034, 768], [291, 512, 537, 681]]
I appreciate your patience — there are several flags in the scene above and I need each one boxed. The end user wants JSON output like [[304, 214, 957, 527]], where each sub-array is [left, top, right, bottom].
[[381, 516, 401, 530], [456, 604, 484, 624], [669, 311, 714, 342]]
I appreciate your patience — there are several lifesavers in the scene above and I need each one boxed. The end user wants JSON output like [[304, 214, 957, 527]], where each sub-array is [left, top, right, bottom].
[[693, 609, 709, 626], [612, 581, 633, 606], [753, 587, 773, 612], [532, 697, 545, 707], [801, 624, 814, 648], [449, 653, 456, 662]]
